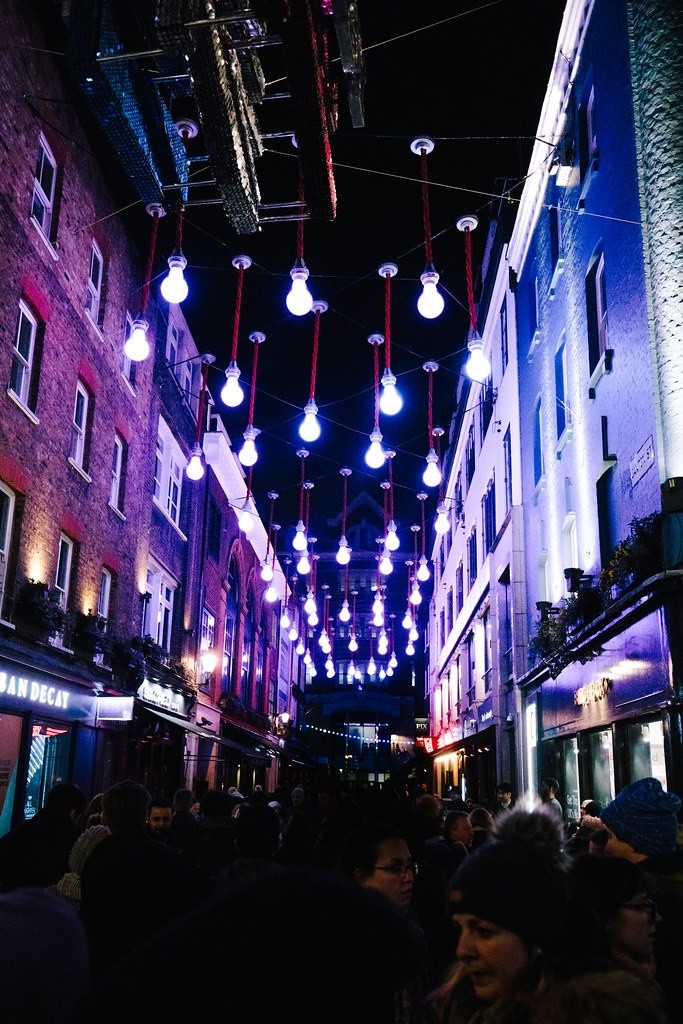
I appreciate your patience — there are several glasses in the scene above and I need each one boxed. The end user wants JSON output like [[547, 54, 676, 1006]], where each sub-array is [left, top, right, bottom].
[[372, 862, 419, 877], [618, 899, 657, 920]]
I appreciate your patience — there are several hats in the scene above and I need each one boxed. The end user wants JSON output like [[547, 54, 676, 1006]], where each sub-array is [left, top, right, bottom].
[[0, 887, 90, 1024], [563, 852, 651, 941], [446, 810, 566, 950], [268, 801, 281, 809], [228, 787, 244, 799], [600, 777, 681, 856]]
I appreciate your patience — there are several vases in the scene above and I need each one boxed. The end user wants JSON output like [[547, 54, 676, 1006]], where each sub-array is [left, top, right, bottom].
[[605, 566, 638, 607]]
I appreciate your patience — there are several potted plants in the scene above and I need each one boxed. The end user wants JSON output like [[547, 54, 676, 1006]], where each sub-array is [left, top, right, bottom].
[[627, 512, 661, 581], [216, 691, 272, 732], [117, 633, 189, 682], [557, 589, 601, 634], [19, 576, 69, 633], [75, 608, 116, 654], [527, 618, 563, 654]]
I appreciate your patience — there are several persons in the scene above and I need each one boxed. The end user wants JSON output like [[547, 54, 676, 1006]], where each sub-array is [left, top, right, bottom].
[[0, 774, 683, 1024]]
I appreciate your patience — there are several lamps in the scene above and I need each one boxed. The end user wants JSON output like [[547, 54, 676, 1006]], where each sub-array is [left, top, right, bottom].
[[161, 118, 199, 304], [421, 360, 444, 489], [185, 354, 216, 477], [562, 567, 596, 592], [286, 160, 315, 317], [377, 262, 405, 415], [432, 424, 452, 536], [411, 137, 447, 319], [236, 329, 268, 468], [298, 299, 329, 442], [457, 212, 493, 381], [139, 591, 153, 604], [273, 707, 291, 736], [536, 600, 560, 622], [185, 628, 196, 638], [198, 671, 212, 685], [234, 447, 450, 680], [121, 203, 166, 363], [218, 255, 254, 406]]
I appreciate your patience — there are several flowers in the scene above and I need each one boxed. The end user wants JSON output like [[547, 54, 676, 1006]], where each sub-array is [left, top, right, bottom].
[[599, 538, 637, 586]]
